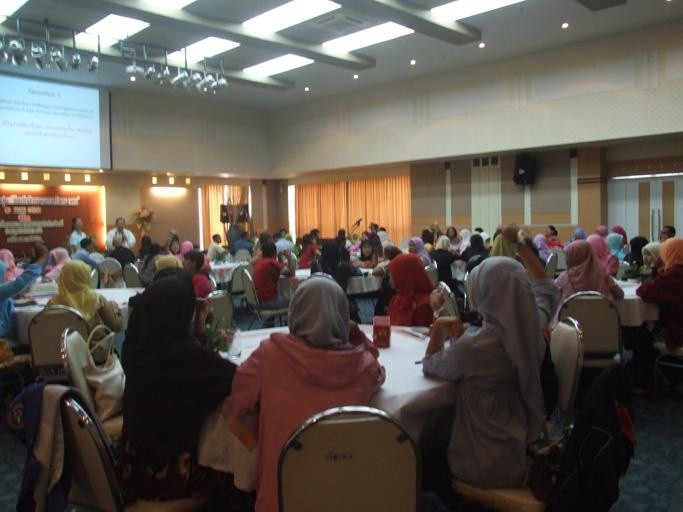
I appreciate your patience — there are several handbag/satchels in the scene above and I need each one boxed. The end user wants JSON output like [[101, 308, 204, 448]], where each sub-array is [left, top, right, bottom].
[[522, 423, 632, 512], [82, 324, 126, 422]]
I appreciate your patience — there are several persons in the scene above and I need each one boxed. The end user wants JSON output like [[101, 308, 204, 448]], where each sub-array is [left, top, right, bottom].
[[385, 222, 683, 398], [423, 257, 558, 512], [1, 212, 385, 512]]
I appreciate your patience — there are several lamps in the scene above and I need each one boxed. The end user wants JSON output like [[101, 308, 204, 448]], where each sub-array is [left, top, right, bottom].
[[0, 21, 230, 91]]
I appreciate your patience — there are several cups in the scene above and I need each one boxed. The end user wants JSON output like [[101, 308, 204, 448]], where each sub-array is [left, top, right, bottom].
[[226, 336, 241, 359], [372, 325, 390, 347]]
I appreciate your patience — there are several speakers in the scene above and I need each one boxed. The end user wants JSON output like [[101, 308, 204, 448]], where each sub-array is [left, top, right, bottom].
[[513, 154, 534, 185]]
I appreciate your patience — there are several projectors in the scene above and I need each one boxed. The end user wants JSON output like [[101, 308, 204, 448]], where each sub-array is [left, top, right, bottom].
[[125, 65, 145, 75]]
